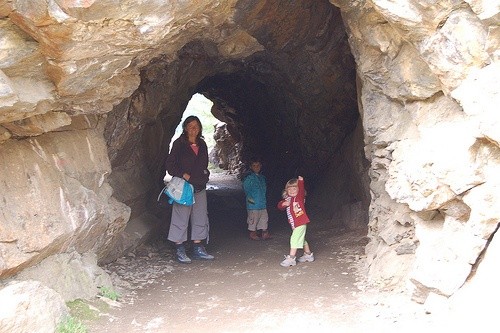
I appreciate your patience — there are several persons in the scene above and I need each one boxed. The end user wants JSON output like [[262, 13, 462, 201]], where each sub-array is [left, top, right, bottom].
[[277, 175, 315, 268], [165, 116, 216, 264], [242, 158, 273, 241]]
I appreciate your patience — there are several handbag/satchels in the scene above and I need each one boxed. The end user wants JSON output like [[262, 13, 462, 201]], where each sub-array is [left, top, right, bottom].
[[164, 176, 195, 207]]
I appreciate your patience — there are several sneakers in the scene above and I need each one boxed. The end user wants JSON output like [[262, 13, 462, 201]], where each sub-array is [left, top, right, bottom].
[[176, 247, 192, 263], [193, 246, 214, 260], [280, 255, 296, 266], [298, 251, 314, 262]]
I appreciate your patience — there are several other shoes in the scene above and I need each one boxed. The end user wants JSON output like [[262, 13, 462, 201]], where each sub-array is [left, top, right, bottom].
[[250, 232, 259, 240], [262, 233, 272, 240]]
